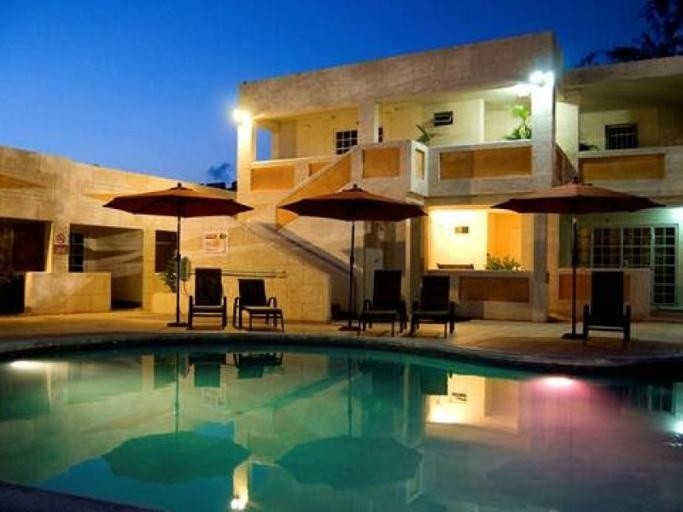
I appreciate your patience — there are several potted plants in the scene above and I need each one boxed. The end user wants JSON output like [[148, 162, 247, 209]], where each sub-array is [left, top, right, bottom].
[[151, 257, 190, 313]]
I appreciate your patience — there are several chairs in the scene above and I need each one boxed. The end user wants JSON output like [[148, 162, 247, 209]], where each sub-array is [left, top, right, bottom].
[[408, 276, 455, 338], [582, 271, 630, 351], [187, 350, 226, 388], [188, 267, 226, 329], [411, 364, 453, 395], [358, 361, 406, 379], [233, 279, 283, 332], [233, 353, 282, 377], [356, 269, 407, 335]]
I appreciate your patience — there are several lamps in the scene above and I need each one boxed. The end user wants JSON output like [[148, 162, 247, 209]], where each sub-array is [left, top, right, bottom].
[[455, 226, 468, 234]]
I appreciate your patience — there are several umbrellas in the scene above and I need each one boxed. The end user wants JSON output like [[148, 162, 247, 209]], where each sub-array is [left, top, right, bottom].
[[273, 356, 423, 492], [484, 395, 659, 511], [488, 175, 668, 335], [99, 350, 252, 486], [276, 181, 430, 327], [100, 180, 256, 324]]
